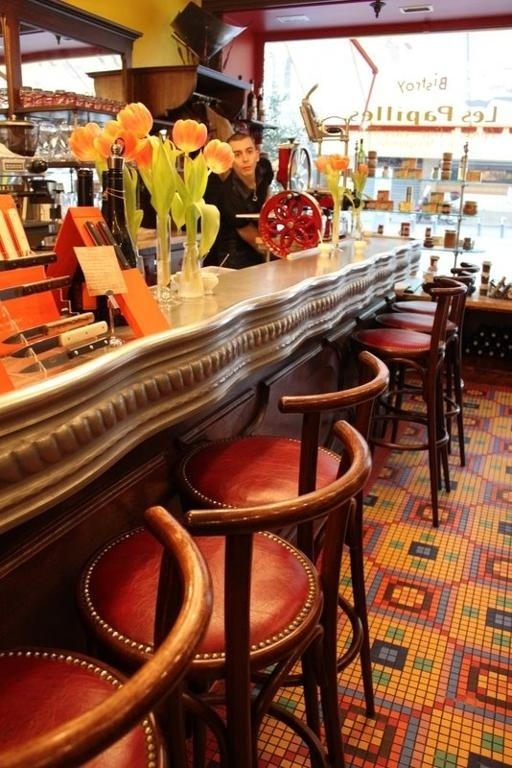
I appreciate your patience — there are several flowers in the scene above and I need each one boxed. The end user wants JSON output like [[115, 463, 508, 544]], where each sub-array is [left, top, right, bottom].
[[68, 99, 235, 283], [313, 154, 369, 241]]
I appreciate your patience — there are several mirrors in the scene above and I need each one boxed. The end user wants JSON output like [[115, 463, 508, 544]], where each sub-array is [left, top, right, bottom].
[[0, 1, 146, 207]]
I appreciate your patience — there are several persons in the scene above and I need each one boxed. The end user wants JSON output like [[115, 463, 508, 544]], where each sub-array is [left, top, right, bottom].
[[200, 132, 275, 270]]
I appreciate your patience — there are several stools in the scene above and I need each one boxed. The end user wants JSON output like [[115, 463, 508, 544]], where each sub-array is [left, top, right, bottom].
[[1, 504, 214, 768], [323, 277, 468, 528], [375, 296, 465, 491], [77, 418, 372, 768], [390, 294, 470, 455], [176, 350, 390, 740]]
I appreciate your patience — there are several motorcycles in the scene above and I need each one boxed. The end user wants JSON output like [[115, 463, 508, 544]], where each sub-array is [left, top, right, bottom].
[[414, 185, 463, 225]]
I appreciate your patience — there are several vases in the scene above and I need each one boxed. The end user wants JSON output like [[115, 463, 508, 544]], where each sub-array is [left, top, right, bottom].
[[120, 213, 205, 311]]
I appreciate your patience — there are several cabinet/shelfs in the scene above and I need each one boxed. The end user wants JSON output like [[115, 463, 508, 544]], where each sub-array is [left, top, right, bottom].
[[347, 140, 511, 275]]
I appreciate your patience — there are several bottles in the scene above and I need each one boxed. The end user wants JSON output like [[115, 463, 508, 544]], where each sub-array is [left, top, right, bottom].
[[445, 229, 471, 250], [463, 200, 477, 215], [320, 208, 348, 241], [236, 73, 265, 122], [179, 242, 203, 299], [77, 144, 137, 269], [457, 157, 468, 182], [356, 139, 366, 177]]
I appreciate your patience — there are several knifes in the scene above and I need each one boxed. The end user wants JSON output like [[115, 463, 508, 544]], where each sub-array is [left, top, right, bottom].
[[0, 251, 112, 373]]
